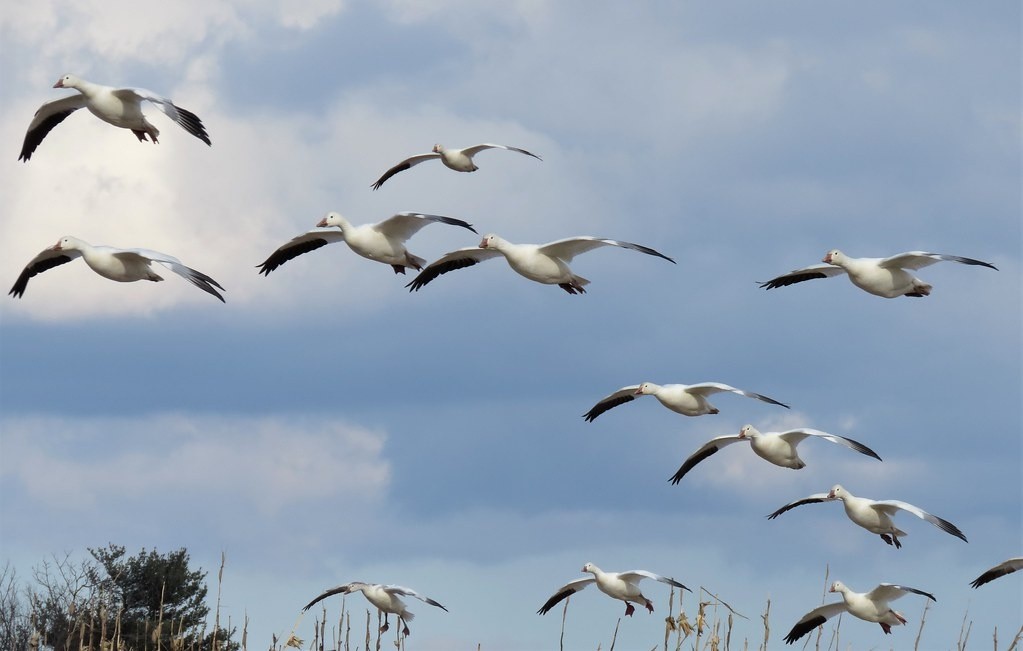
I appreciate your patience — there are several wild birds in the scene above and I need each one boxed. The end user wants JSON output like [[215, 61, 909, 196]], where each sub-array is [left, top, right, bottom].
[[667, 424, 883, 485], [782, 580, 937, 645], [8, 235, 227, 304], [755, 247, 999, 299], [255, 212, 480, 278], [764, 484, 968, 550], [18, 74, 213, 163], [404, 233, 677, 296], [581, 381, 791, 424], [301, 581, 449, 638], [536, 562, 694, 618], [968, 558, 1023, 590], [369, 141, 544, 191]]
